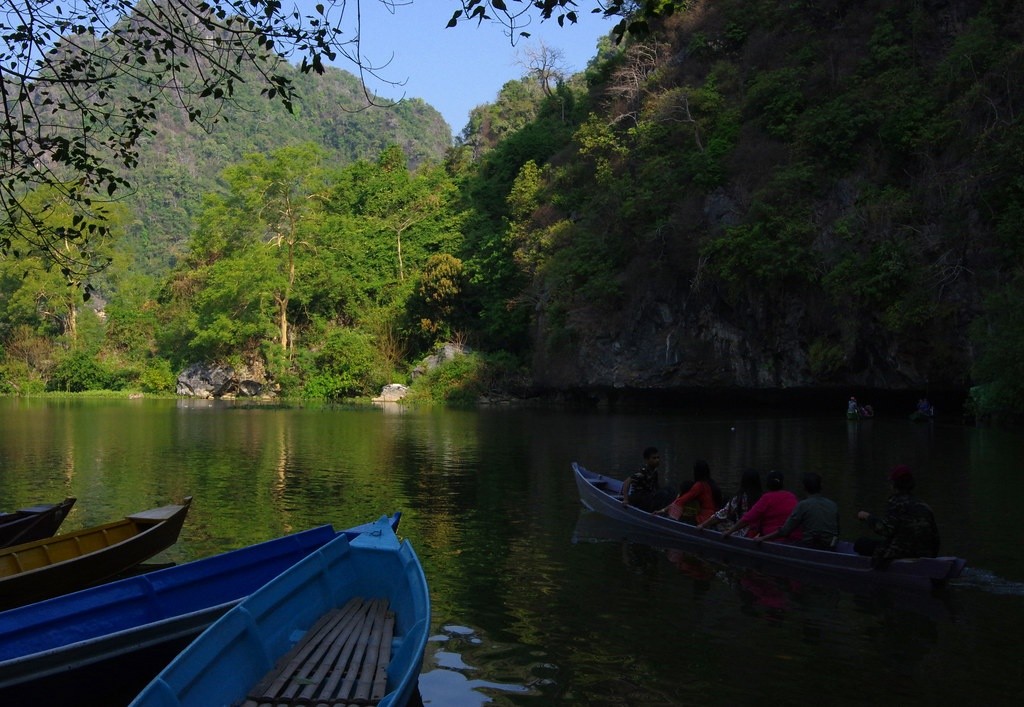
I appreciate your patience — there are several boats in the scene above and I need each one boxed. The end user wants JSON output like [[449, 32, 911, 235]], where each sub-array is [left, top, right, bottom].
[[570, 507, 965, 625], [0, 494, 194, 610], [1, 512, 403, 704], [567, 462, 968, 594], [126, 515, 432, 707], [0, 496, 75, 548]]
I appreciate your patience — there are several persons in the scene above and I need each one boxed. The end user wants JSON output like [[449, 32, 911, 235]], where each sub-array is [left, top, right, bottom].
[[679, 481, 700, 522], [622, 447, 676, 511], [917, 398, 929, 410], [753, 471, 840, 552], [722, 471, 803, 546], [652, 459, 722, 528], [868, 463, 936, 570], [696, 468, 764, 537], [621, 526, 802, 630], [848, 397, 857, 413]]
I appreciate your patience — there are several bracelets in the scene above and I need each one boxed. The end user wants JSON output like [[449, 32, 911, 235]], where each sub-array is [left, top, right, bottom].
[[734, 525, 739, 530]]
